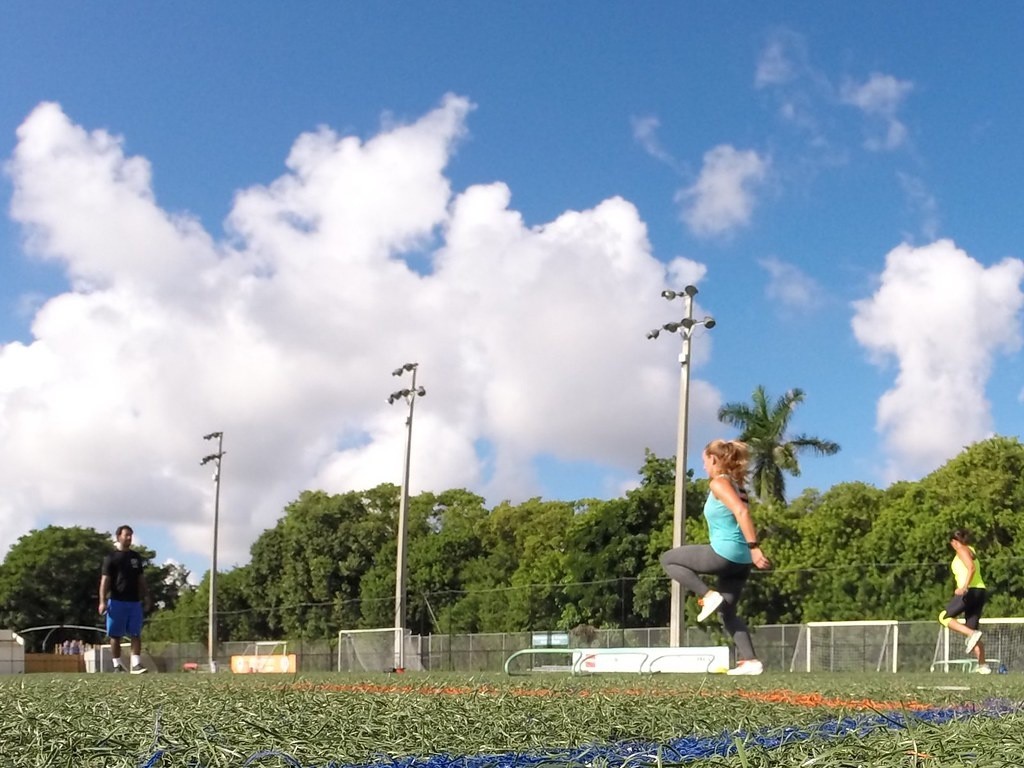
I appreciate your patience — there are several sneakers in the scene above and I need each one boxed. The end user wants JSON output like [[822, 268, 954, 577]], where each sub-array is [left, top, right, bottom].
[[726, 659, 764, 676], [696, 590, 724, 622], [970, 663, 993, 675], [963, 628, 983, 655], [129, 664, 148, 675], [111, 664, 129, 674]]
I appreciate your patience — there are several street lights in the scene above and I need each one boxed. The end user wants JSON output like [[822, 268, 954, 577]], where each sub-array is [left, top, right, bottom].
[[198, 430, 228, 671], [646, 284, 716, 648], [388, 363, 427, 673]]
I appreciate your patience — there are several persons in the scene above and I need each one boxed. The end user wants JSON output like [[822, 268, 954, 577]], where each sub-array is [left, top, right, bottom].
[[938, 530, 992, 676], [99, 525, 149, 675], [658, 439, 773, 678]]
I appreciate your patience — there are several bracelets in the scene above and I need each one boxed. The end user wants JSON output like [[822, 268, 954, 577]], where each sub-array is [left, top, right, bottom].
[[747, 542, 760, 548], [963, 585, 969, 593]]
